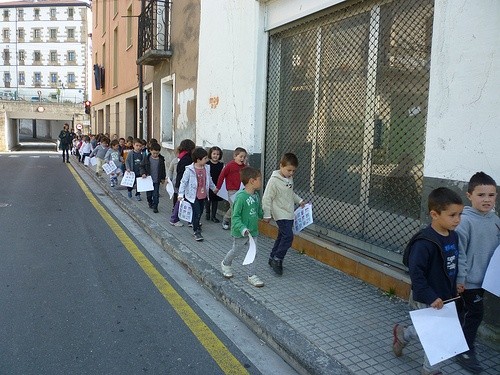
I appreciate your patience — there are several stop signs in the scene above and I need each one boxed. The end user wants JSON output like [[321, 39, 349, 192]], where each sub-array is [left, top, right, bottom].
[[76, 124, 83, 130]]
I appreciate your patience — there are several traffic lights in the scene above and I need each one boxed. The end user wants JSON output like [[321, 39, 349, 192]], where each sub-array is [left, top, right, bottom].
[[85, 101, 90, 114]]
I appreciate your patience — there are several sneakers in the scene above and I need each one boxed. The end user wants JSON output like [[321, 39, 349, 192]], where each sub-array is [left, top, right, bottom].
[[392, 324, 407, 357], [452, 345, 484, 374], [420, 368, 442, 375]]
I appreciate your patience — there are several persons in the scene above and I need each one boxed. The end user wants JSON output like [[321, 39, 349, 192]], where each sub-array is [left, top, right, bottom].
[[69, 127, 307, 288], [393, 172, 500, 375], [59, 123, 71, 164]]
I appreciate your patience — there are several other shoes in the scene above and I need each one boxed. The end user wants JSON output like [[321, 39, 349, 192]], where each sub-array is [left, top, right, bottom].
[[128, 191, 132, 198], [110, 182, 114, 187], [187, 223, 193, 227], [247, 275, 264, 287], [193, 226, 203, 241], [114, 179, 118, 184], [220, 260, 235, 278], [152, 206, 159, 213], [136, 194, 141, 201], [170, 220, 184, 227], [206, 217, 219, 223], [269, 256, 284, 275], [149, 203, 153, 209], [221, 222, 230, 230]]
